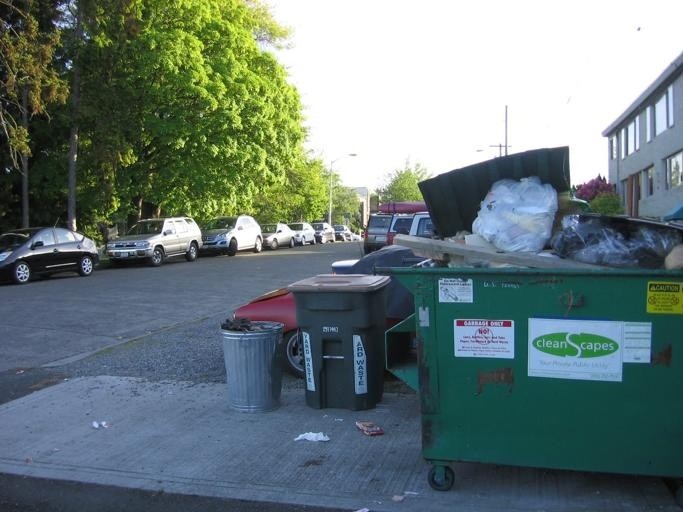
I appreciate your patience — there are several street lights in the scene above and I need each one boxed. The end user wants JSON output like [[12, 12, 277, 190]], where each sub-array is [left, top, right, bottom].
[[327, 153, 356, 225]]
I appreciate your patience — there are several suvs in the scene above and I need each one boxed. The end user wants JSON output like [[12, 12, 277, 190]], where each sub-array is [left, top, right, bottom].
[[200, 214, 263, 256], [105, 217, 203, 266], [363, 212, 438, 254]]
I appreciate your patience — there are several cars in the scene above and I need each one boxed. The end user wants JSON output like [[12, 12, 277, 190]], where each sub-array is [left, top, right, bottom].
[[231, 244, 430, 378], [0, 226, 100, 284], [259, 222, 360, 250]]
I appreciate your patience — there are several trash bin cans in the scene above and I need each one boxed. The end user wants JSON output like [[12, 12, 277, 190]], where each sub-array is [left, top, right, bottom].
[[287, 273, 392, 412], [374, 258, 683, 491], [219, 319, 285, 413]]
